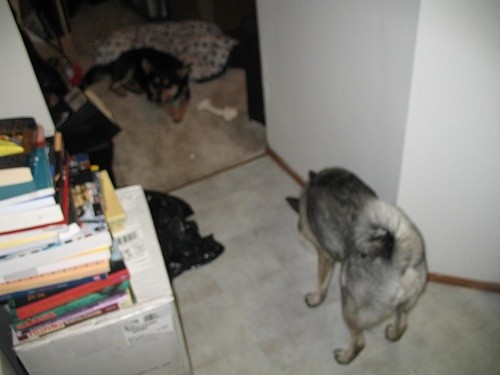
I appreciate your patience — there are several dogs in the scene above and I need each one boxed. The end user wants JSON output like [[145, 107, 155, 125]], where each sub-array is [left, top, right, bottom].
[[286, 167, 431, 366], [78, 44, 194, 124]]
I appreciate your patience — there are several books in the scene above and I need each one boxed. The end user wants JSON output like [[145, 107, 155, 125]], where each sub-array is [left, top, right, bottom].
[[0, 116, 136, 341]]
